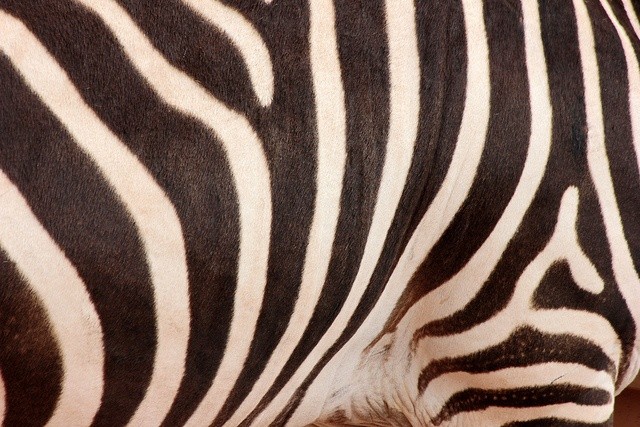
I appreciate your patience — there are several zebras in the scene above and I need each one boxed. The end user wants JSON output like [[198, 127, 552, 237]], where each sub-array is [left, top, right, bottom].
[[0, 0, 640, 427]]
